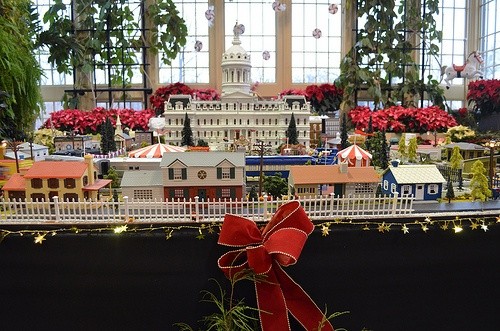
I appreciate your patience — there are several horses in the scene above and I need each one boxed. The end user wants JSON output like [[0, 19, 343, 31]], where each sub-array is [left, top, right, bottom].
[[441, 50, 485, 90]]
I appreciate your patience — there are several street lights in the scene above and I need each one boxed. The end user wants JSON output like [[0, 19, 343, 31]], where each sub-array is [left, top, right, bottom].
[[483, 140, 500, 189]]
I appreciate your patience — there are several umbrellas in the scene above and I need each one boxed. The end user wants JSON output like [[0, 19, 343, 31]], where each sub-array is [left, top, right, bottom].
[[126, 135, 187, 158]]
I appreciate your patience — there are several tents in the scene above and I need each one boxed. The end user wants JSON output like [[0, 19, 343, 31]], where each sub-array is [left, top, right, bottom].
[[334, 144, 373, 167]]
